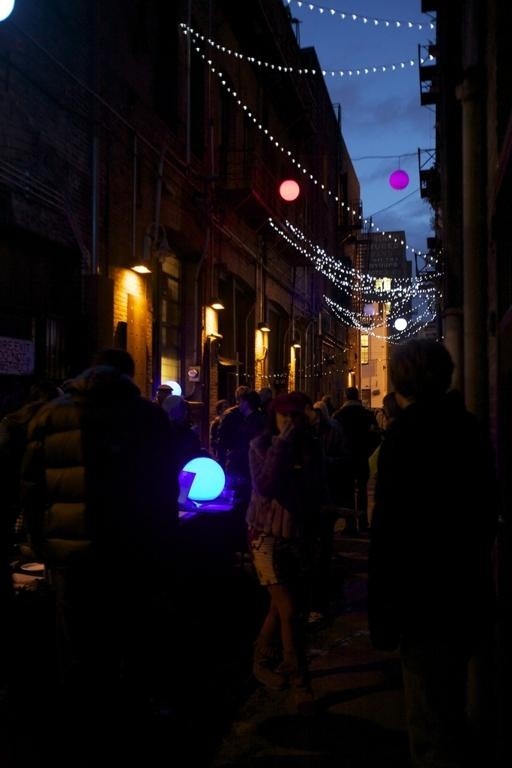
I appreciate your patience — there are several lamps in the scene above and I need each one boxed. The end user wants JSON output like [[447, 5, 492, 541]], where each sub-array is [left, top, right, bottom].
[[128, 253, 301, 348]]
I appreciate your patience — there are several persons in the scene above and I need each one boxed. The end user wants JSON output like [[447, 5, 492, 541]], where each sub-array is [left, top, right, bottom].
[[1, 337, 512, 768]]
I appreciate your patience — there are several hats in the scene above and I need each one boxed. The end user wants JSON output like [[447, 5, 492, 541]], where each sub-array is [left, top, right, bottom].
[[266, 393, 305, 415], [388, 338, 455, 399]]
